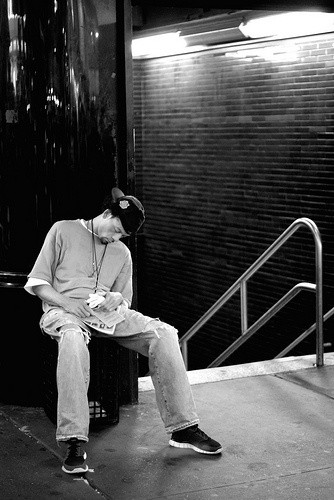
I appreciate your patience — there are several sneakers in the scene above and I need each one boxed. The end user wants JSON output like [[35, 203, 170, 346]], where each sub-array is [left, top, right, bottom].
[[61, 438, 90, 475], [170, 423, 224, 456]]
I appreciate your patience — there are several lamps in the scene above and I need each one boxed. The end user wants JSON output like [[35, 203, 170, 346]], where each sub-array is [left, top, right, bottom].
[[130, 10, 333, 60]]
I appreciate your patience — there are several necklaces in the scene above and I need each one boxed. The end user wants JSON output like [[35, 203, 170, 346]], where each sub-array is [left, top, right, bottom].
[[90, 219, 107, 295]]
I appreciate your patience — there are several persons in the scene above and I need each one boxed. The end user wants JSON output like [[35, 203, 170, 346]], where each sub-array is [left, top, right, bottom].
[[22, 187, 223, 474]]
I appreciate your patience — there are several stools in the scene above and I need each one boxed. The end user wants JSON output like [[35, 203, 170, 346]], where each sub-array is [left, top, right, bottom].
[[40, 329, 120, 435]]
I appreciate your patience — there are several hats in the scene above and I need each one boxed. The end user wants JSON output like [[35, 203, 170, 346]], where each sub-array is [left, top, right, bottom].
[[112, 187, 145, 238]]
